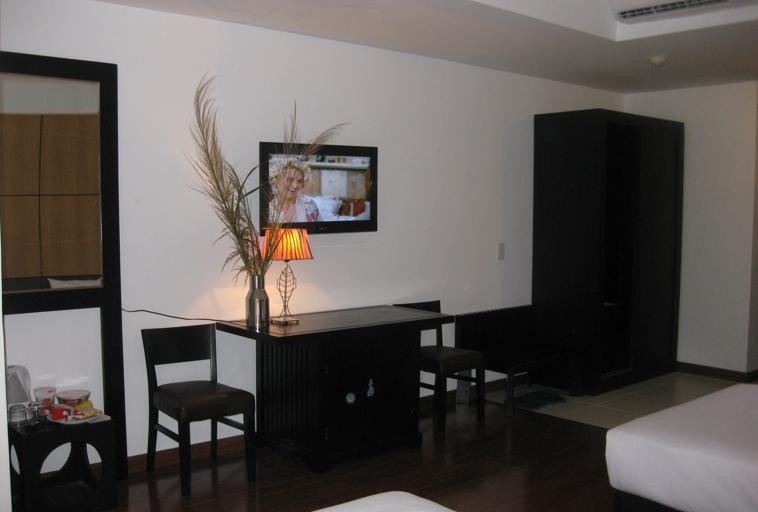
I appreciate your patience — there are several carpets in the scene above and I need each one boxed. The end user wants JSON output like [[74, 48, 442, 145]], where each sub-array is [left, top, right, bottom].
[[475, 371, 740, 429]]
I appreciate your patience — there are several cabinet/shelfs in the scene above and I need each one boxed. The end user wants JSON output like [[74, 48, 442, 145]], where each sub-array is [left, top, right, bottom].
[[0, 113, 101, 278], [531, 108, 684, 397]]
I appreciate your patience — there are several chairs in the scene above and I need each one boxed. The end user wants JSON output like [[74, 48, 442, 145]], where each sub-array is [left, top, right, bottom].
[[141, 323, 256, 497], [393, 300, 485, 432]]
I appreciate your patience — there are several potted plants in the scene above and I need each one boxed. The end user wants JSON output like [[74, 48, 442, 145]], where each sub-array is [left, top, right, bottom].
[[186, 74, 350, 326]]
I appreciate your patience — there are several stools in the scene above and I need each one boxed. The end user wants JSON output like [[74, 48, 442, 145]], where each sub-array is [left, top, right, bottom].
[[462, 340, 552, 418]]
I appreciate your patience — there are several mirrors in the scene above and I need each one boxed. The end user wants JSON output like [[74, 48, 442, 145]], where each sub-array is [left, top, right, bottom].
[[0, 51, 119, 315]]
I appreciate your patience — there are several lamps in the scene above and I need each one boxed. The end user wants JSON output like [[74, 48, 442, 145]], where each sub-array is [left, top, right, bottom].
[[261, 229, 314, 325]]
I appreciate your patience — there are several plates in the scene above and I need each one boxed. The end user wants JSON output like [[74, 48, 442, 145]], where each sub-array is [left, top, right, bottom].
[[47, 409, 103, 425]]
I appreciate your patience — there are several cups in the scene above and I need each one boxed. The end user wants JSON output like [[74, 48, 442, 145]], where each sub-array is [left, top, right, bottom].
[[33, 386, 56, 416], [56, 389, 91, 418], [7, 401, 41, 432]]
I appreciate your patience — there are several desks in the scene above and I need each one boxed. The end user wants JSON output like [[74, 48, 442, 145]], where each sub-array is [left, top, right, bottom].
[[8, 414, 118, 512], [213, 305, 454, 474]]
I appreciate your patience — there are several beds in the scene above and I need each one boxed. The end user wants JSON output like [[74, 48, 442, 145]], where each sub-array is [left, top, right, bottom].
[[605, 382, 758, 512]]
